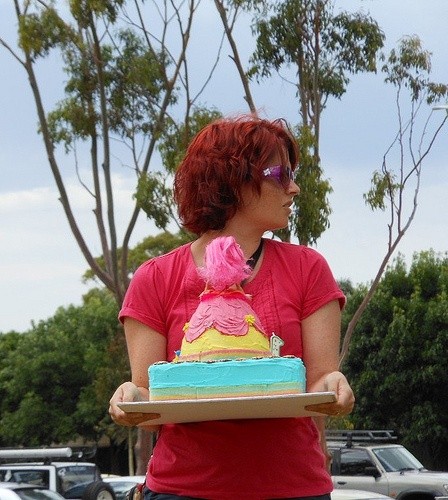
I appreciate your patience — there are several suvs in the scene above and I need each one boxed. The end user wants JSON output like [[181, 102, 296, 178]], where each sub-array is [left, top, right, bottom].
[[323, 431, 448, 500], [0, 448, 116, 500]]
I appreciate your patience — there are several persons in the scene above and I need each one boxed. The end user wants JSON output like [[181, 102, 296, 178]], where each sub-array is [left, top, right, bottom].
[[104, 115, 358, 500]]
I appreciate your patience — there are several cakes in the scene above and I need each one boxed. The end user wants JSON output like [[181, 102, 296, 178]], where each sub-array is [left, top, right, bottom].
[[147, 236, 307, 400]]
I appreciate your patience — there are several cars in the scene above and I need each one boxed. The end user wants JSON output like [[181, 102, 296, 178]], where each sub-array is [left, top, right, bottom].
[[0, 483, 67, 500], [330, 489, 395, 500]]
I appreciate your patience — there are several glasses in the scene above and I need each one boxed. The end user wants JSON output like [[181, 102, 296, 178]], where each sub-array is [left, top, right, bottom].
[[261, 165, 295, 191]]
